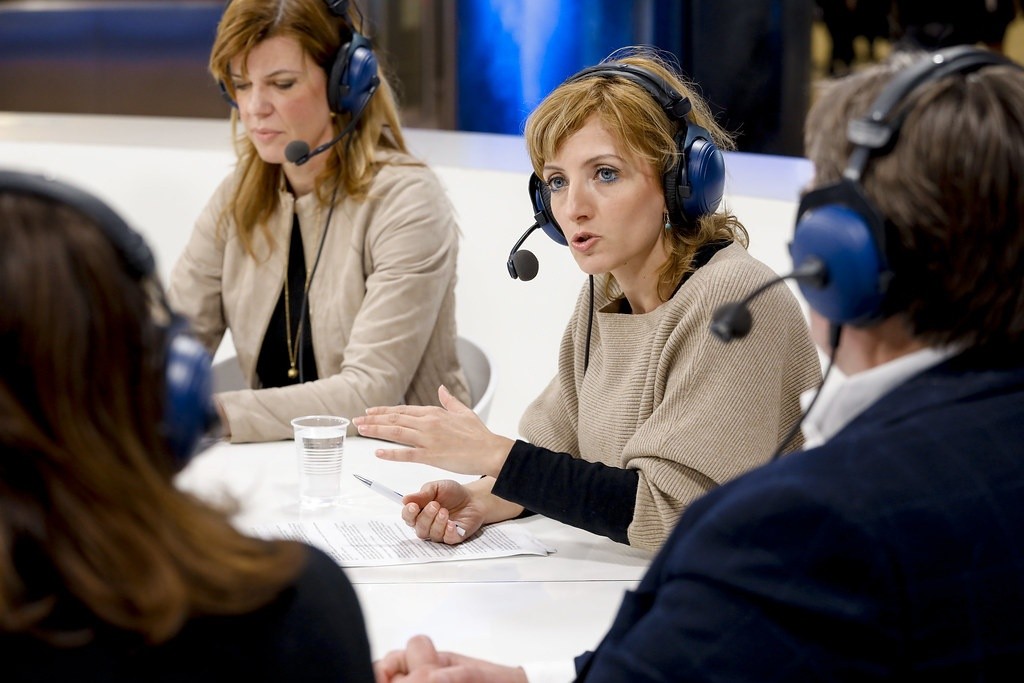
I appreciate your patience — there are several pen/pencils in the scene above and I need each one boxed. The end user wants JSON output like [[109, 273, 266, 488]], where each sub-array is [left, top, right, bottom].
[[353, 474, 466, 536]]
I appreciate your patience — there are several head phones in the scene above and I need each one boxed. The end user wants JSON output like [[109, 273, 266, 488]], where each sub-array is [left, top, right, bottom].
[[527, 62, 725, 247], [219, 0, 381, 116], [792, 44, 1024, 348], [0, 168, 226, 473]]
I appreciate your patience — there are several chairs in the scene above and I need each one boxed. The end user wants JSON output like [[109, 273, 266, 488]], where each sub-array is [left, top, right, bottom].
[[450, 333, 495, 428]]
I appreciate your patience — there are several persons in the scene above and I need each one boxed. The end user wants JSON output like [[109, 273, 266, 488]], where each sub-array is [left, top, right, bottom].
[[374, 43, 1024, 683], [162, 0, 473, 446], [0, 170, 374, 683], [349, 58, 823, 556]]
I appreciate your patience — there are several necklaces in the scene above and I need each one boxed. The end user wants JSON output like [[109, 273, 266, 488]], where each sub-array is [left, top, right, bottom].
[[281, 204, 326, 378]]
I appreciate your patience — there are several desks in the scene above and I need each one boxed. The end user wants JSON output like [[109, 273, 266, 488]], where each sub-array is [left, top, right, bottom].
[[164, 424, 648, 683]]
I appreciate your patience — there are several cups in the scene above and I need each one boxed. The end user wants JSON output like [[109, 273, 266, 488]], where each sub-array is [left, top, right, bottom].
[[291, 416, 350, 505]]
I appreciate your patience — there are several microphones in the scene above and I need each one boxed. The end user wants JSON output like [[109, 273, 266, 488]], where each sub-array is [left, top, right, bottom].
[[284, 87, 375, 165], [507, 222, 539, 282], [713, 271, 799, 344]]
